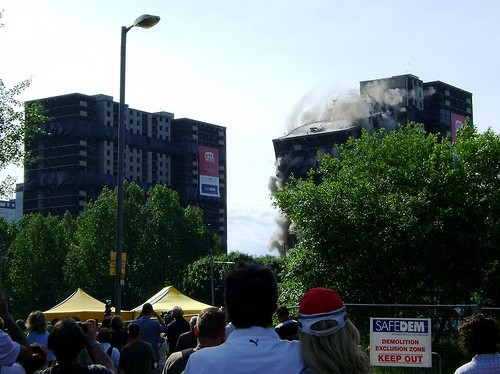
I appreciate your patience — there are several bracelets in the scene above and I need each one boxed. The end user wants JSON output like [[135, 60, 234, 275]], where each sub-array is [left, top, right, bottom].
[[89, 341, 99, 351], [1, 311, 13, 324]]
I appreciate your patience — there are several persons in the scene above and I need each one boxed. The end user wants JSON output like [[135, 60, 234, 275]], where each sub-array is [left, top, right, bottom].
[[0, 296, 235, 374], [274, 306, 299, 341], [452, 314, 500, 374], [298, 287, 372, 374], [182, 259, 306, 374]]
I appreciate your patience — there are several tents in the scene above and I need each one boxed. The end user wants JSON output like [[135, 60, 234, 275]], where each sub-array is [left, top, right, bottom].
[[130, 286, 222, 326], [42, 286, 134, 322]]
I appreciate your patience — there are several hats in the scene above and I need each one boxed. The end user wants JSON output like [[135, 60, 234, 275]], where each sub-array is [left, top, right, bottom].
[[295, 287, 347, 336], [127, 321, 141, 334]]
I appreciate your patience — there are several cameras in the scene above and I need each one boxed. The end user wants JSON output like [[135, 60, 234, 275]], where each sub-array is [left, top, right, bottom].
[[76, 322, 89, 334]]
